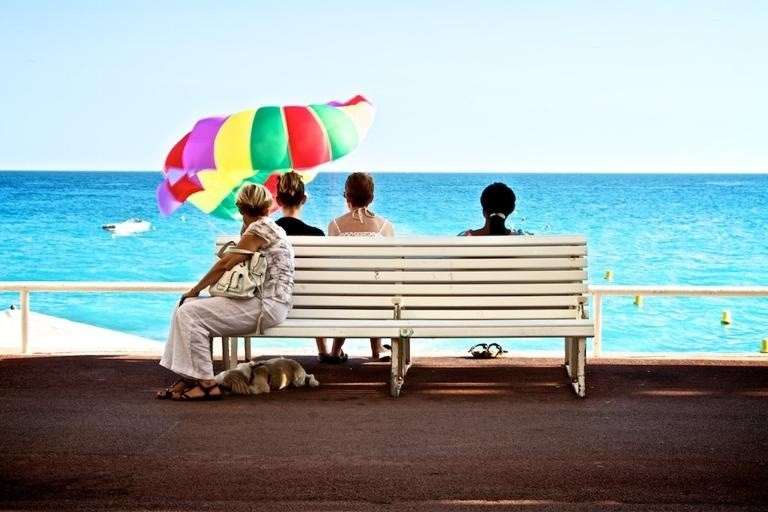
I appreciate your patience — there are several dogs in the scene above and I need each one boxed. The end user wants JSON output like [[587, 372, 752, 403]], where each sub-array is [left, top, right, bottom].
[[213, 355, 321, 397]]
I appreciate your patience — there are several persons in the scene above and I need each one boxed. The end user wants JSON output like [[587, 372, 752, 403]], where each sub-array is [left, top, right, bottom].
[[157, 183, 295, 402], [275, 171, 329, 362], [328, 172, 394, 363], [457, 182, 535, 236]]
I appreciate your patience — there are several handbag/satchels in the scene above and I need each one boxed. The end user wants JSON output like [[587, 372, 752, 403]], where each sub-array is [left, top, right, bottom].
[[209, 242, 268, 300]]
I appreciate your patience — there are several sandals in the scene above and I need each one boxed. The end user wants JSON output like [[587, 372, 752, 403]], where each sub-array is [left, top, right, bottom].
[[469, 342, 508, 358], [155, 376, 225, 402], [318, 348, 348, 364]]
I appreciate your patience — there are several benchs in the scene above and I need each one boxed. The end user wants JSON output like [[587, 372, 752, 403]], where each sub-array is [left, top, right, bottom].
[[215, 235, 594, 398]]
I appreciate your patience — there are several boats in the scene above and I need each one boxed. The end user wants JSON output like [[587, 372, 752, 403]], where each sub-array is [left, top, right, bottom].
[[103, 218, 152, 231]]
